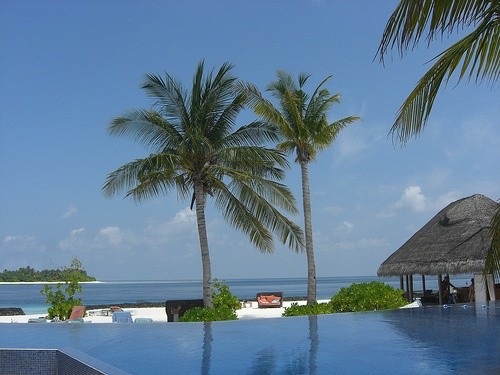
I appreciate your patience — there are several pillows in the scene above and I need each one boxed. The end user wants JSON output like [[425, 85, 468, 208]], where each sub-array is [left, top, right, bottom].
[[266, 295, 274, 303], [274, 297, 281, 301], [260, 296, 266, 302]]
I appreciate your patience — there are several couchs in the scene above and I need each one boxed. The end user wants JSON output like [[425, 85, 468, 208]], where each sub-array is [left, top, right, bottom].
[[257, 292, 282, 307]]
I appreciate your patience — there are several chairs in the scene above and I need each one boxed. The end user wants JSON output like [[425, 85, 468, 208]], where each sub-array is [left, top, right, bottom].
[[60, 306, 85, 323]]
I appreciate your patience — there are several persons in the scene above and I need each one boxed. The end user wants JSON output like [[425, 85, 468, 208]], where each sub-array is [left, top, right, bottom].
[[468, 277, 475, 302], [439, 275, 456, 305]]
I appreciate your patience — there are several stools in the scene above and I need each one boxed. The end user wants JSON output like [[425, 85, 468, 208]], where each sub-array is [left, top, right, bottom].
[[240, 302, 244, 307], [102, 310, 108, 316]]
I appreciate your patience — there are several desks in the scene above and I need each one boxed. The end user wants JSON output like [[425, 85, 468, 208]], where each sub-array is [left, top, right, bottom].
[[112, 312, 133, 323], [134, 318, 152, 323]]
